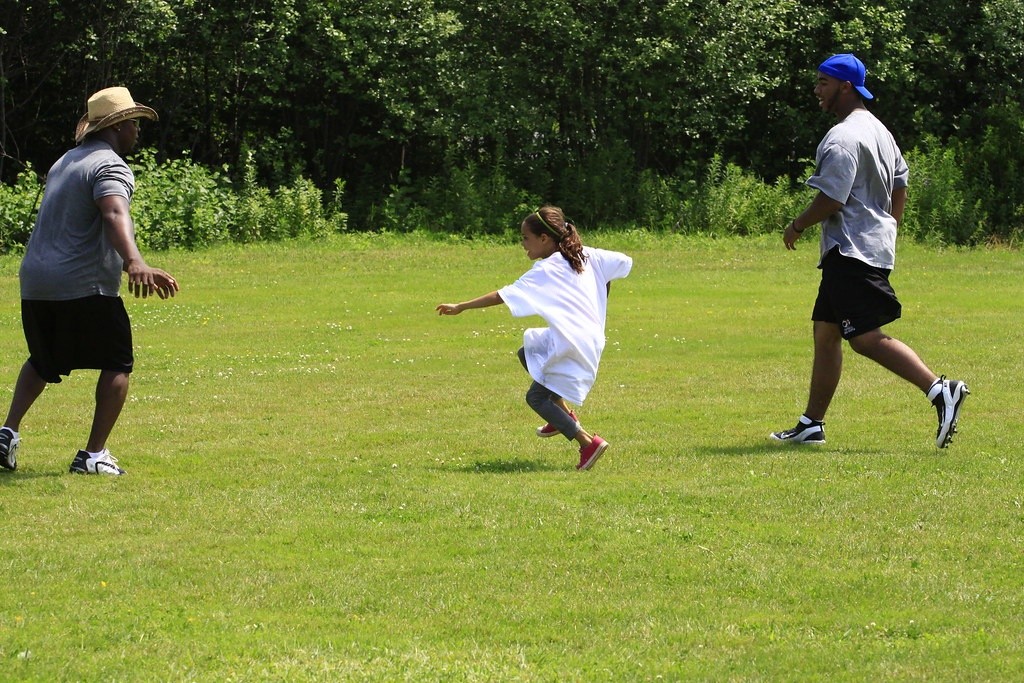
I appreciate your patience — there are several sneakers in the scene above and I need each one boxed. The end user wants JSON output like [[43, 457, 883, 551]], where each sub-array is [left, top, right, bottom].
[[536, 411, 578, 437], [770, 415, 827, 445], [577, 432, 609, 472], [69, 447, 128, 475], [0, 426, 20, 470], [926, 375, 972, 448]]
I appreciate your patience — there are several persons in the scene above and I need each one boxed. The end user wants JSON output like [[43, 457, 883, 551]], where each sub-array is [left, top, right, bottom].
[[437, 206, 635, 472], [0, 87, 183, 481], [771, 52, 973, 450]]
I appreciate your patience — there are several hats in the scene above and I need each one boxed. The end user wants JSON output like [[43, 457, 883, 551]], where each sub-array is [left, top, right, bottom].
[[75, 87, 158, 142], [817, 53, 874, 100]]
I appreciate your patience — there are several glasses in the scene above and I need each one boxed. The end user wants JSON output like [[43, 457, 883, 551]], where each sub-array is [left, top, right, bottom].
[[126, 117, 141, 126]]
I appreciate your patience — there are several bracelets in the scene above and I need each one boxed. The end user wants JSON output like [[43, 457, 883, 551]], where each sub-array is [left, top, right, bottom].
[[791, 218, 804, 234]]
